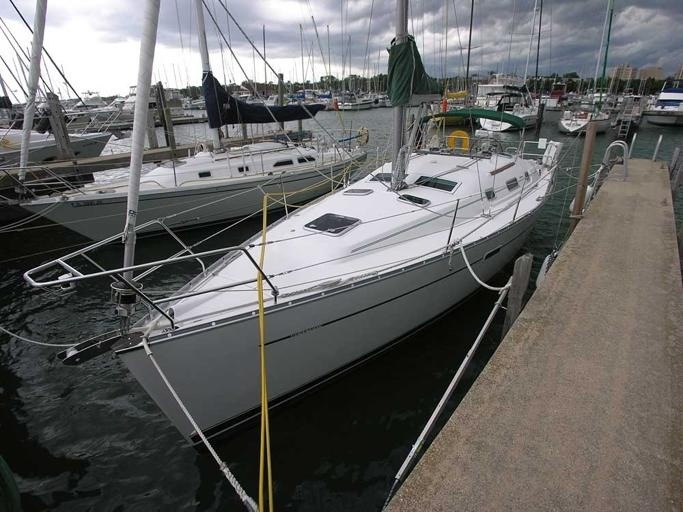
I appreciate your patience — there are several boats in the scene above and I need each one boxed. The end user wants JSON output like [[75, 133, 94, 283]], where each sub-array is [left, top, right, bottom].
[[647, 78, 683, 127]]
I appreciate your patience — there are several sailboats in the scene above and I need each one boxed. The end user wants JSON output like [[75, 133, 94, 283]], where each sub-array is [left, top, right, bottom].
[[529, 66, 667, 109], [0, 0, 124, 192], [557, 0, 617, 136], [0, 0, 372, 247], [478, 0, 546, 132], [21, 1, 568, 455], [60, 0, 442, 132], [427, 1, 476, 126]]
